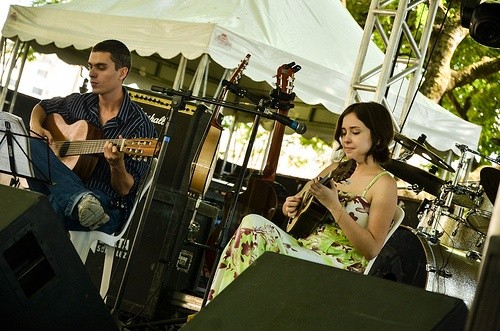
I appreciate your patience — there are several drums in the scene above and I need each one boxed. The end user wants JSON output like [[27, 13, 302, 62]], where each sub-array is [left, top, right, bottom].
[[364, 225, 483, 312], [464, 188, 493, 235], [416, 208, 487, 257]]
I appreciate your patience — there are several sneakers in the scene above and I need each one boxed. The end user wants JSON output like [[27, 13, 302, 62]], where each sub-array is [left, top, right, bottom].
[[77, 195, 110, 231]]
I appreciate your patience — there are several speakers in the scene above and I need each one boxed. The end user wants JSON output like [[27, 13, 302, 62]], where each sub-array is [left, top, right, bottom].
[[123, 86, 223, 197], [173, 249, 468, 331], [0, 184, 121, 331]]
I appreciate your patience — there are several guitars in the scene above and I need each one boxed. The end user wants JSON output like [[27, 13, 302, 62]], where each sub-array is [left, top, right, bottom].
[[38, 113, 161, 171], [189, 53, 252, 194], [206, 62, 302, 275], [288, 158, 358, 238]]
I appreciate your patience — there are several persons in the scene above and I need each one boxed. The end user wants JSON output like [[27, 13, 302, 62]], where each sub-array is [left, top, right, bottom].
[[207, 101, 398, 304], [29, 39, 155, 238]]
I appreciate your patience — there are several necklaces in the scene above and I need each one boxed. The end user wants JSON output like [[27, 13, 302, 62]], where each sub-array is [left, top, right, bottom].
[[345, 181, 350, 185]]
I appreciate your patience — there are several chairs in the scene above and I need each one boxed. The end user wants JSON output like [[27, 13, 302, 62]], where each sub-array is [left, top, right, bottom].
[[69, 158, 158, 304]]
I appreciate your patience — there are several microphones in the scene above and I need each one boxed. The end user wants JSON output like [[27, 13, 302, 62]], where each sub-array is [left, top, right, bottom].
[[259, 107, 308, 134]]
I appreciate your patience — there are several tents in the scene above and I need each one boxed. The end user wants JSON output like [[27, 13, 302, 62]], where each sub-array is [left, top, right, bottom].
[[0, 0, 483, 196]]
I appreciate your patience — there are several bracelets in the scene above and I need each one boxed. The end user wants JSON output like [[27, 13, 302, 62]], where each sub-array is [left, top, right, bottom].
[[335, 206, 343, 223]]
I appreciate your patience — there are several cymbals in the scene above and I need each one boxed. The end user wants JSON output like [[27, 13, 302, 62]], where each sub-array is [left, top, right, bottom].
[[392, 129, 458, 172], [381, 158, 451, 199]]
